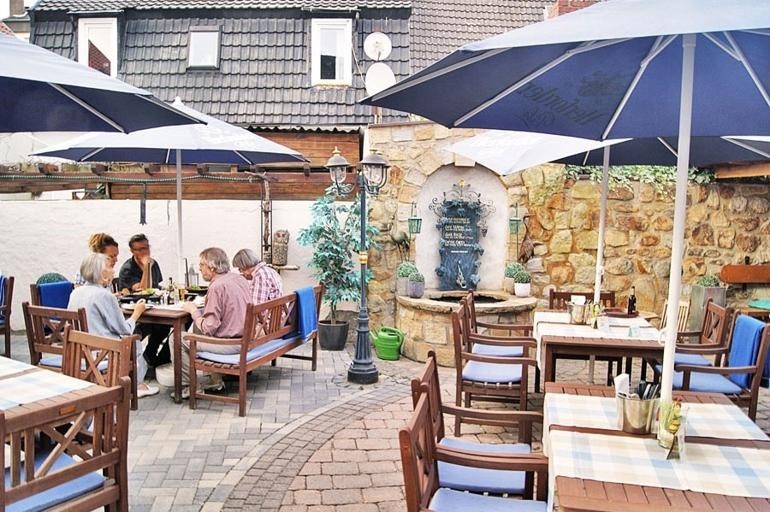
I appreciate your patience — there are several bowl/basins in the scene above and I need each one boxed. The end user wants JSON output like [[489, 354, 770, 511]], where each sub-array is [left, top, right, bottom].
[[119, 290, 163, 302], [186, 287, 209, 295]]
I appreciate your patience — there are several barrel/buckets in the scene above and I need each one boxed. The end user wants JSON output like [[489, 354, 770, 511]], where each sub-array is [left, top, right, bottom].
[[566, 301, 591, 324], [616, 393, 660, 436]]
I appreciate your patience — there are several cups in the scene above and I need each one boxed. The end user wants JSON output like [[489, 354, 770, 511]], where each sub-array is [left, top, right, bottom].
[[652, 401, 692, 452], [178, 286, 185, 300], [588, 304, 606, 329], [184, 294, 198, 301]]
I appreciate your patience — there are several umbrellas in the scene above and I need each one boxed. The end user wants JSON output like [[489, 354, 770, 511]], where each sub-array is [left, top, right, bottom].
[[27, 96, 311, 282], [0, 31, 208, 135], [358, 1, 770, 420], [441, 130, 770, 383]]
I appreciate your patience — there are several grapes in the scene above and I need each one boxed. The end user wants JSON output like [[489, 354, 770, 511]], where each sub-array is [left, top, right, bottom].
[[187, 285, 201, 291]]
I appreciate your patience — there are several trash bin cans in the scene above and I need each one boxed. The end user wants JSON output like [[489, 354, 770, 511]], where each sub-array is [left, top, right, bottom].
[[747, 299, 770, 388]]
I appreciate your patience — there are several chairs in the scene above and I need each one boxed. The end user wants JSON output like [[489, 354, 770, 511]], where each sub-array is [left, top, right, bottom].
[[547, 289, 618, 310], [398, 381, 550, 511], [667, 308, 770, 423], [461, 289, 537, 408], [660, 297, 694, 342], [669, 295, 732, 367], [448, 299, 539, 444], [0, 276, 137, 512], [408, 347, 550, 498]]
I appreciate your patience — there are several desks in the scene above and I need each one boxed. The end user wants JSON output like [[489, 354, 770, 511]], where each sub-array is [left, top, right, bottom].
[[536, 288, 668, 386], [542, 377, 769, 512]]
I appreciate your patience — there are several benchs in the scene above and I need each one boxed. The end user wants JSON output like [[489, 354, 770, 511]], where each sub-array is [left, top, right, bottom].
[[183, 284, 325, 415]]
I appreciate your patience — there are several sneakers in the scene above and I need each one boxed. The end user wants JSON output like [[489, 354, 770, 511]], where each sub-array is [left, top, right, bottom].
[[171, 381, 225, 399]]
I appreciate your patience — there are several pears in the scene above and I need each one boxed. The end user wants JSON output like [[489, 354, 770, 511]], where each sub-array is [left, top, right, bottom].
[[146, 287, 154, 294]]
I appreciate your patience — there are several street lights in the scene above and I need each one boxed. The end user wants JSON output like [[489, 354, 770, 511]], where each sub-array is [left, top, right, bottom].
[[326, 144, 391, 385]]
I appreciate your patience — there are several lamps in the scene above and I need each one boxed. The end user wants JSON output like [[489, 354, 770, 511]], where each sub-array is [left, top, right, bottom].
[[510, 202, 521, 261], [407, 200, 422, 260]]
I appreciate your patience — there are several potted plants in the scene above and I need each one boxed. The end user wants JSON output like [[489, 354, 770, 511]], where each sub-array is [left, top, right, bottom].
[[396, 262, 418, 295], [298, 187, 378, 351], [504, 263, 524, 293], [408, 272, 424, 297], [513, 270, 531, 297]]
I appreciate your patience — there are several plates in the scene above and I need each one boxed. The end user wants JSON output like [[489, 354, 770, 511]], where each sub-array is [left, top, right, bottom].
[[123, 304, 152, 311]]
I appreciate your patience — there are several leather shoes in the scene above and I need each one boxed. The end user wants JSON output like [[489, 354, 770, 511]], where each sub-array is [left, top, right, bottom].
[[137, 383, 159, 398]]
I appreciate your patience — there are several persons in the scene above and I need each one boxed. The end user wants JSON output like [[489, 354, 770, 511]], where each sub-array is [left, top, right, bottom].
[[232, 249, 284, 336], [169, 246, 252, 400], [65, 253, 160, 399], [117, 235, 194, 365], [73, 232, 123, 298]]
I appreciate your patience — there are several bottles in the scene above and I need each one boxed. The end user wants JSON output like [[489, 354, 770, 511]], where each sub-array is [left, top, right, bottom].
[[628, 287, 637, 313], [167, 277, 175, 304]]
